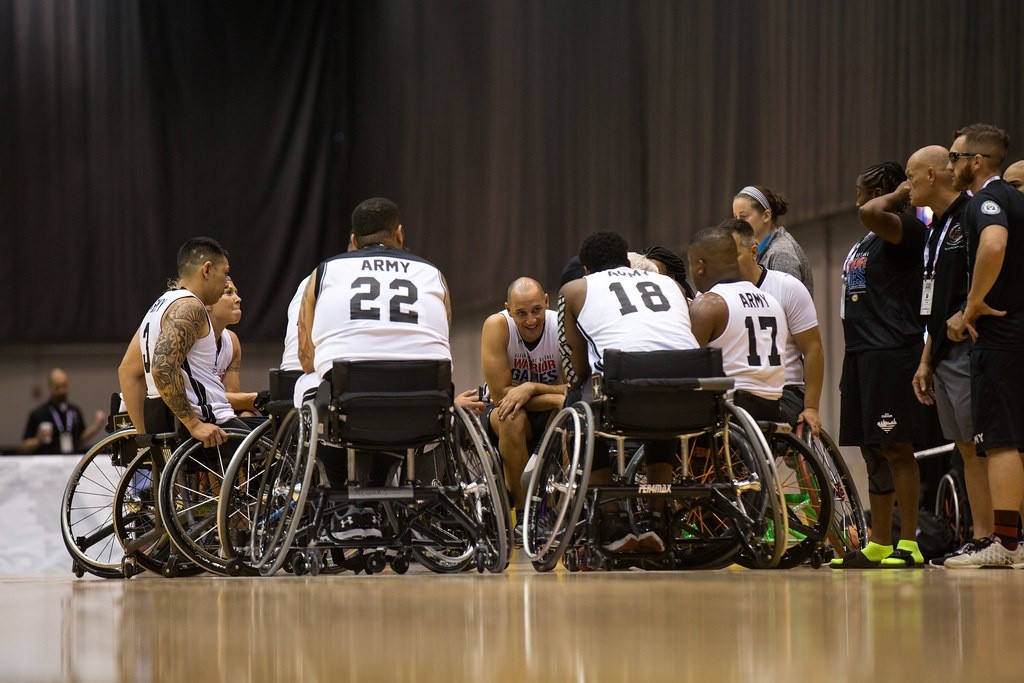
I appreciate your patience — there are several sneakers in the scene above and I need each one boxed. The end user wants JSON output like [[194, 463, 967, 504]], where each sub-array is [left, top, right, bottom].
[[943, 534, 1024, 570], [570, 509, 638, 553], [930, 536, 994, 569], [514, 506, 567, 536], [634, 508, 675, 553], [319, 506, 363, 541], [360, 507, 381, 537]]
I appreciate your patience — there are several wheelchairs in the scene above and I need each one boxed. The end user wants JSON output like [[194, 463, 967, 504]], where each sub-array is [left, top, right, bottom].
[[59, 346, 868, 580]]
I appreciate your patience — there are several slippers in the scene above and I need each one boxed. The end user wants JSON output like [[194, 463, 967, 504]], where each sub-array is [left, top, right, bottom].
[[878, 548, 925, 569], [829, 550, 880, 569]]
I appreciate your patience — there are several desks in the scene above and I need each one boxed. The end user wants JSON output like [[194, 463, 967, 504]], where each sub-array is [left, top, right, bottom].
[[0, 452, 136, 578]]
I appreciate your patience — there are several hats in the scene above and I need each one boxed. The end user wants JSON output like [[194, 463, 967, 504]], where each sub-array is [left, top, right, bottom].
[[559, 254, 587, 289], [627, 251, 659, 274]]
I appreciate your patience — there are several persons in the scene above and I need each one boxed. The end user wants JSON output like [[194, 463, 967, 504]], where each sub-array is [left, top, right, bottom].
[[117, 125, 1024, 570], [22, 369, 107, 454]]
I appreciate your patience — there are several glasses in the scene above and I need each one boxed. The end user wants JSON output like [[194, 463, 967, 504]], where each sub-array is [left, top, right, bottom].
[[949, 151, 991, 163]]
